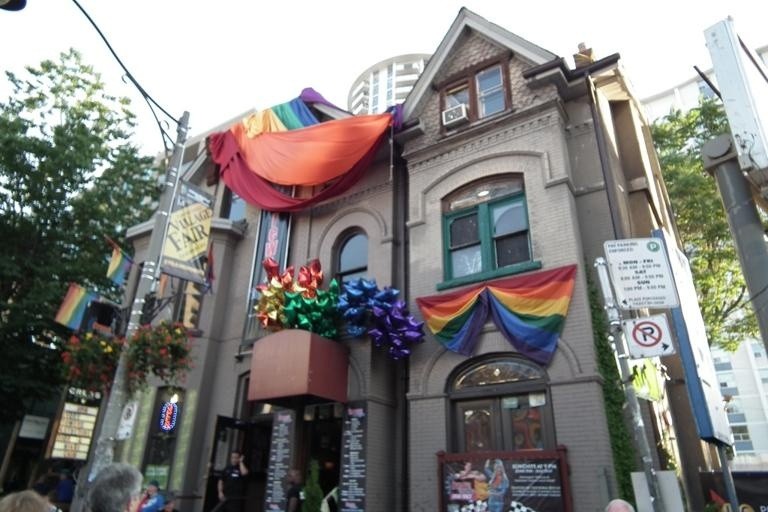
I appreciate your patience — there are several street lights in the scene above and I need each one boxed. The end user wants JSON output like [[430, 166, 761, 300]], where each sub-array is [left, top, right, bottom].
[[1, 1, 191, 487]]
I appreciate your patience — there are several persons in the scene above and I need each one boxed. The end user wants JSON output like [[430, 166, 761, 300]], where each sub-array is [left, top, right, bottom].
[[479, 458, 511, 512], [281, 468, 308, 512], [2, 460, 181, 512], [209, 447, 255, 511]]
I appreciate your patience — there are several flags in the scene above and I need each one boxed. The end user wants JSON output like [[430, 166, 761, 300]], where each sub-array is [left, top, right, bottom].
[[51, 282, 102, 331], [106, 236, 132, 287]]
